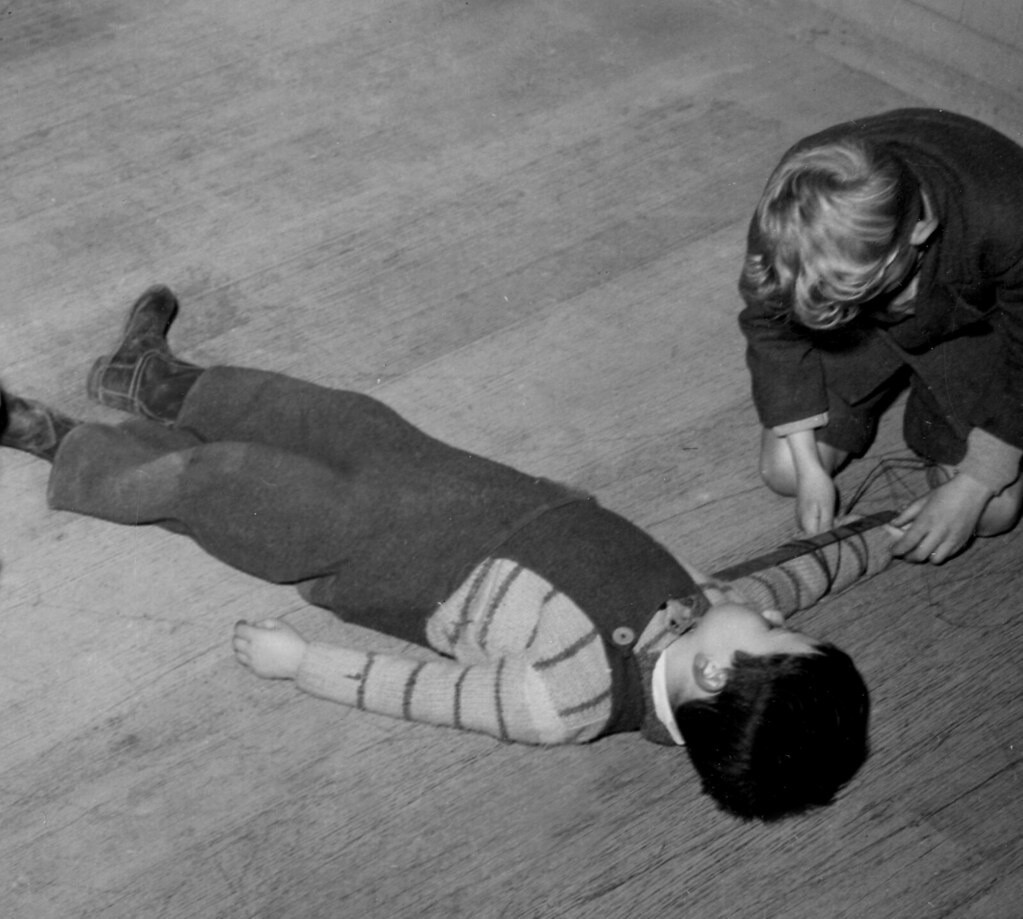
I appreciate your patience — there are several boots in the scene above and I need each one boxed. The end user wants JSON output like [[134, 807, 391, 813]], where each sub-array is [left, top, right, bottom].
[[0, 388, 84, 464], [86, 285, 205, 430]]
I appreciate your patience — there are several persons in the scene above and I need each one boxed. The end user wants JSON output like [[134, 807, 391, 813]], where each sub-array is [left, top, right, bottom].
[[0, 282, 911, 824], [736, 108, 1023, 565]]
